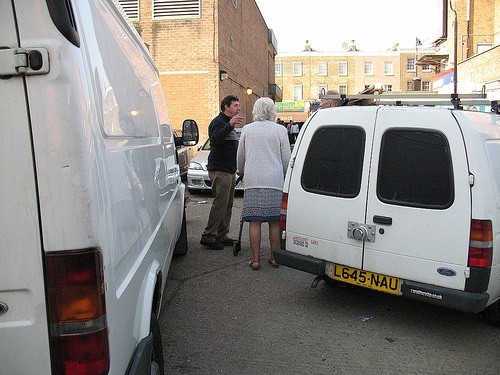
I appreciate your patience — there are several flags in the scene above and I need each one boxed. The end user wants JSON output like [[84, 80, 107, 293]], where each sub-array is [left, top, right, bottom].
[[416, 37, 422, 46]]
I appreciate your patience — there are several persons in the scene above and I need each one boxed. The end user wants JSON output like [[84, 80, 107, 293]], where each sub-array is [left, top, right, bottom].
[[200, 96, 246, 249], [237, 97, 291, 271], [319, 90, 339, 109], [277, 117, 301, 145]]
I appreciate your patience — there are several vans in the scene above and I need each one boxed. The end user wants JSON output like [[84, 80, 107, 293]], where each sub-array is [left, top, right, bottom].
[[0, 0, 200, 375], [273, 92, 500, 328]]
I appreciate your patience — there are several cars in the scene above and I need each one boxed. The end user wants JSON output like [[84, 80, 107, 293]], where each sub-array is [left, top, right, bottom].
[[186, 127, 245, 195]]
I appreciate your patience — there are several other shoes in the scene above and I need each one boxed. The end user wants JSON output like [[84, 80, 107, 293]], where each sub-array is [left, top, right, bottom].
[[200, 238, 225, 250], [222, 238, 237, 246]]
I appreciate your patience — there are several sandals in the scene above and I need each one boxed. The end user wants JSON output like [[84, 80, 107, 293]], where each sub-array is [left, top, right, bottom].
[[249, 259, 260, 270], [269, 259, 279, 268]]
